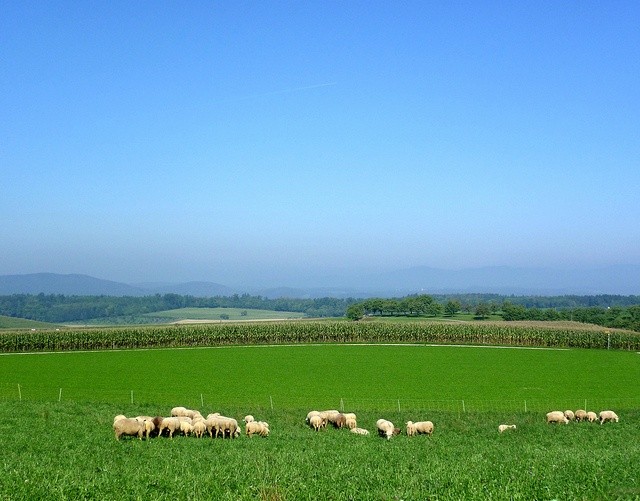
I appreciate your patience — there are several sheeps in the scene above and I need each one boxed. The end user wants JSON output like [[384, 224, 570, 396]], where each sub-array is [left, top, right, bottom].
[[251, 420, 269, 429], [405, 421, 418, 437], [327, 413, 346, 428], [305, 411, 321, 425], [349, 428, 369, 435], [112, 407, 242, 442], [574, 409, 588, 421], [310, 415, 322, 431], [587, 411, 599, 423], [245, 423, 270, 438], [394, 428, 402, 435], [598, 410, 619, 425], [547, 414, 570, 425], [346, 418, 357, 429], [318, 409, 340, 428], [343, 413, 356, 421], [563, 410, 576, 421], [546, 410, 564, 417], [242, 415, 254, 424], [414, 421, 434, 436], [498, 424, 516, 434], [375, 418, 394, 441]]
